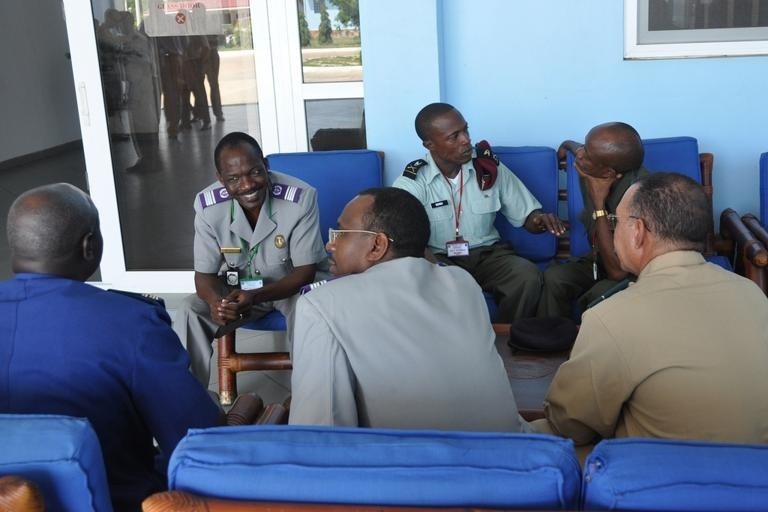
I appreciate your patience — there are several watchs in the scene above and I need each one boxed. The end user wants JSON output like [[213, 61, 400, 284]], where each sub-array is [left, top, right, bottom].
[[592, 209, 608, 221]]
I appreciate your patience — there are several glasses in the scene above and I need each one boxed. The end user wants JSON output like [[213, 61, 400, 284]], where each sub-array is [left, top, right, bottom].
[[328, 227, 394, 246], [607, 213, 651, 232]]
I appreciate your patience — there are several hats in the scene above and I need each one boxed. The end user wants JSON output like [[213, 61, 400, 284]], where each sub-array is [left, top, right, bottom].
[[507, 314, 578, 353], [472, 140, 497, 191]]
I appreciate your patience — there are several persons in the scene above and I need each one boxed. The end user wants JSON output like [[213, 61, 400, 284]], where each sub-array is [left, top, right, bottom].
[[101, 7, 129, 142], [177, 9, 212, 131], [120, 11, 161, 172], [391, 102, 568, 324], [537, 122, 652, 323], [288, 187, 536, 434], [1, 183, 226, 512], [138, 0, 183, 142], [531, 173, 767, 444], [175, 132, 327, 392], [192, 3, 225, 122]]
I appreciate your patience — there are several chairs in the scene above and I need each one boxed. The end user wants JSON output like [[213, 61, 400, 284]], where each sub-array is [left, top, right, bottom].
[[216, 148, 386, 406], [481, 144, 562, 336], [141, 401, 583, 512], [562, 134, 768, 293], [583, 433, 768, 512], [741, 151, 768, 247], [1, 390, 265, 512]]
